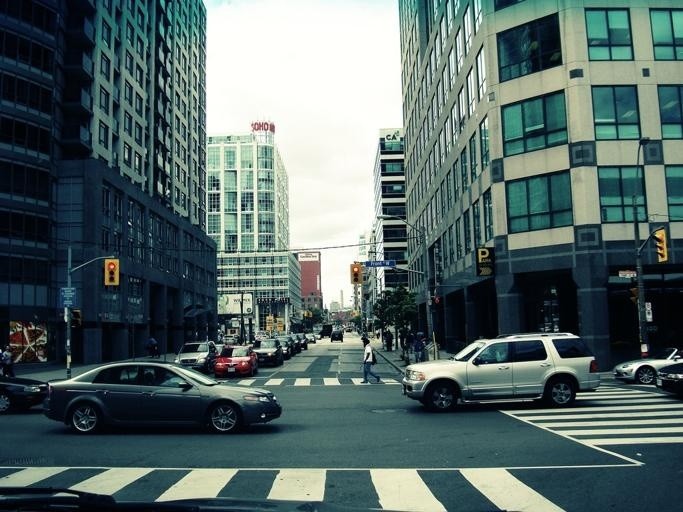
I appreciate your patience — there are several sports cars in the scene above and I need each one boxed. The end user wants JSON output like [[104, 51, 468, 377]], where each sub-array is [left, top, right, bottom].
[[613, 347, 683, 384]]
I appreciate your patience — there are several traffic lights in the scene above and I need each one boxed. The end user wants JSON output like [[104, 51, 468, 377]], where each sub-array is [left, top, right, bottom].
[[651, 227, 668, 262], [70, 310, 82, 328], [307, 311, 311, 317], [629, 287, 638, 304], [350, 264, 363, 284], [104, 259, 119, 287]]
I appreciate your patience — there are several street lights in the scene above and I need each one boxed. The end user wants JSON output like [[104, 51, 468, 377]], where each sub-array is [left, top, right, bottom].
[[376, 212, 434, 342], [631, 135, 652, 358], [240, 289, 245, 342]]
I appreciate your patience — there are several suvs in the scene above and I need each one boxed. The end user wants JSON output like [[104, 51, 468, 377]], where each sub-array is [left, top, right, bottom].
[[251, 339, 284, 367], [330, 330, 342, 342], [400, 329, 600, 415], [221, 334, 239, 345]]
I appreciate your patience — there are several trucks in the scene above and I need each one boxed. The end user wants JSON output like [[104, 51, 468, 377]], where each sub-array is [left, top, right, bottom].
[[312, 324, 332, 338]]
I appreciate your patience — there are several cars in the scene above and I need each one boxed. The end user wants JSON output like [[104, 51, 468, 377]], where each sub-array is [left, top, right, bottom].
[[275, 331, 322, 359], [212, 343, 226, 358], [254, 331, 269, 342], [214, 344, 258, 378], [40, 361, 281, 435], [0, 374, 48, 416], [345, 327, 352, 332], [656, 363, 683, 395]]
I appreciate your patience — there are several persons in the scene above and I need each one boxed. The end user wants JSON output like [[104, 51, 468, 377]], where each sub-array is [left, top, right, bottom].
[[145, 337, 160, 358], [360, 339, 380, 383], [2, 345, 15, 377]]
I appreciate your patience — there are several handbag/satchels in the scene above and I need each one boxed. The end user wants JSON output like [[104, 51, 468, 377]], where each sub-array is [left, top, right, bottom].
[[371, 354, 377, 365]]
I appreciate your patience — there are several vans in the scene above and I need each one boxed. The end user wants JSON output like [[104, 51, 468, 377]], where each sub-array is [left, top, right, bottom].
[[174, 340, 218, 376]]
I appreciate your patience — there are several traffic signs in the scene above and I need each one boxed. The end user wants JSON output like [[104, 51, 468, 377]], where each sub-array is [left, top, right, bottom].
[[366, 259, 395, 267]]
